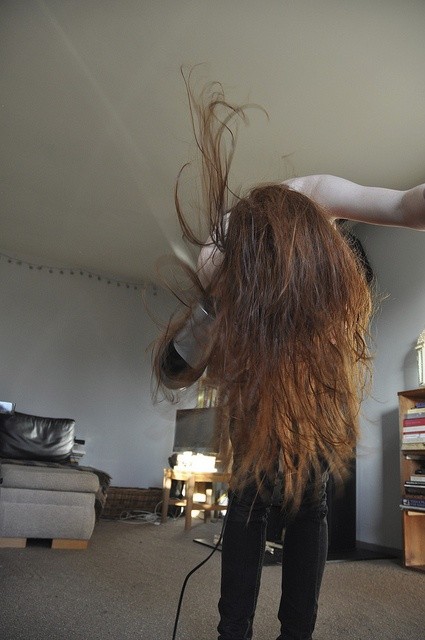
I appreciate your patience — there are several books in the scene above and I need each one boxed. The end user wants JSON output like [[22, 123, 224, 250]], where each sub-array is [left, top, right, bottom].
[[399, 470, 424, 516], [398, 403, 425, 452]]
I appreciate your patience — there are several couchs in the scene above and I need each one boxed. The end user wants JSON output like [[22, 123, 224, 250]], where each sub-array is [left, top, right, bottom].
[[0, 410, 100, 550]]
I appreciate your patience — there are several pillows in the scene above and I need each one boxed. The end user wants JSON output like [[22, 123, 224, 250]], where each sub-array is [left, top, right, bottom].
[[0, 412, 75, 464]]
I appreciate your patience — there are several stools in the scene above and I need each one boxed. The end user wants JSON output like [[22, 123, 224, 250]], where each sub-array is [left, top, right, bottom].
[[158, 467, 230, 531]]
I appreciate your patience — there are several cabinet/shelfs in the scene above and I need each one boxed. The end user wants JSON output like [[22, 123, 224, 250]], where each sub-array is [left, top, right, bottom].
[[397, 386, 425, 572], [267, 441, 357, 562]]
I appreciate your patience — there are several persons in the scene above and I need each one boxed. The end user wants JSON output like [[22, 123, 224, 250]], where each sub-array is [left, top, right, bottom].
[[150, 60, 424, 640]]
